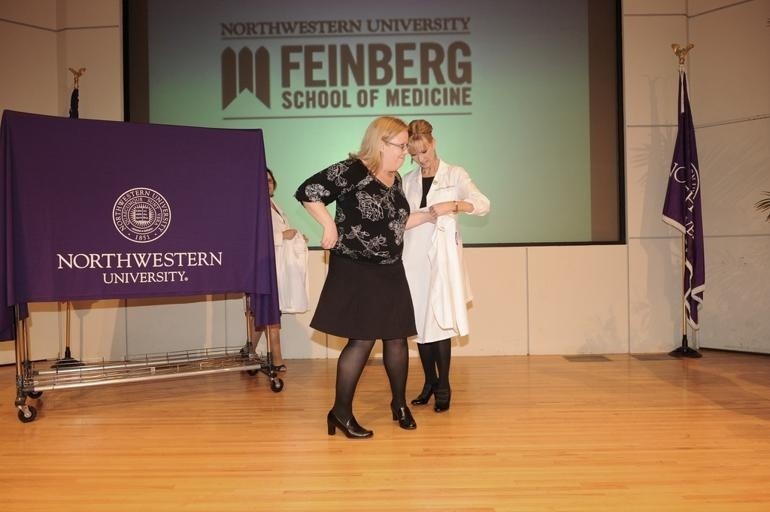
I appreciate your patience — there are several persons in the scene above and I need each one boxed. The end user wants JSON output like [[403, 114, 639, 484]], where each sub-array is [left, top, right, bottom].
[[292, 113, 439, 437], [239, 168, 309, 374], [401, 120, 494, 412]]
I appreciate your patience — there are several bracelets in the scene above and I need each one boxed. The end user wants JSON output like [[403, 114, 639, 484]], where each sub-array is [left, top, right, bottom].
[[452, 200, 459, 213]]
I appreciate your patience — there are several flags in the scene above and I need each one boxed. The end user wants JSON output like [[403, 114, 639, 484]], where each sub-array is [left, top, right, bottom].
[[661, 63, 709, 329], [67, 89, 81, 120]]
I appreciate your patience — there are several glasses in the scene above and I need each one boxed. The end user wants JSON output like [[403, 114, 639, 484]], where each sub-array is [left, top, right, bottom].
[[268, 178, 275, 184], [382, 138, 408, 150]]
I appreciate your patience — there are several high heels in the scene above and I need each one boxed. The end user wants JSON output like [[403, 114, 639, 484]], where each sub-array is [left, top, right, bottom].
[[240, 348, 260, 361], [411, 379, 451, 412], [327, 408, 373, 439], [390, 399, 417, 430], [272, 364, 287, 372]]
